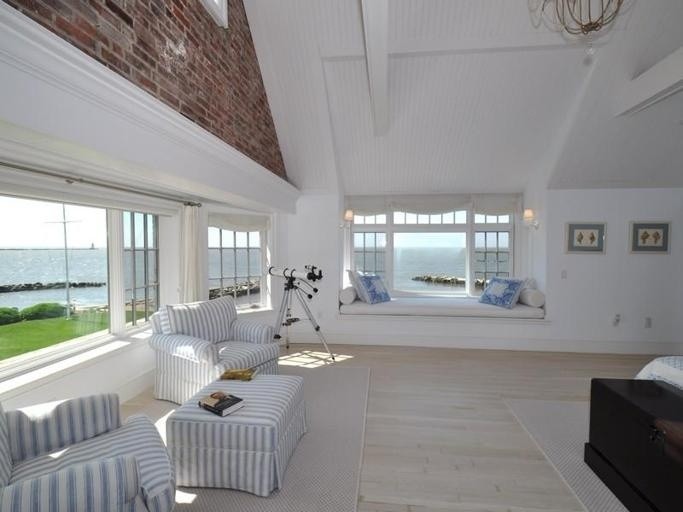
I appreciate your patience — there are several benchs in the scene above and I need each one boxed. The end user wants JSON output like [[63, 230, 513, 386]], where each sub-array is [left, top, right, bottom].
[[164, 372, 309, 498]]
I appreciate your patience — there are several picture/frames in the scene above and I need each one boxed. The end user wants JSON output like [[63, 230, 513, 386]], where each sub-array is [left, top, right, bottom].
[[627, 218, 672, 256], [563, 219, 608, 256]]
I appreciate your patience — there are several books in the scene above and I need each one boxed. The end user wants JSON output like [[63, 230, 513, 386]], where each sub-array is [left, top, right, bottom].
[[220, 367, 258, 381], [199, 391, 245, 416]]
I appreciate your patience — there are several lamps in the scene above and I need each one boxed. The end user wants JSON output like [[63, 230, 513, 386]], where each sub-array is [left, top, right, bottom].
[[338, 210, 356, 232], [539, 0, 624, 56], [523, 208, 542, 233]]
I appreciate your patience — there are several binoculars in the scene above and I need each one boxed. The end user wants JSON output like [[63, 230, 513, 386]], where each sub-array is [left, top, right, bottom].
[[269, 265, 323, 282]]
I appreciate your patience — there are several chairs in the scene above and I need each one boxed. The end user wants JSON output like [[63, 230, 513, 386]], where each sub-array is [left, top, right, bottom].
[[0, 392, 175, 512], [147, 294, 283, 406]]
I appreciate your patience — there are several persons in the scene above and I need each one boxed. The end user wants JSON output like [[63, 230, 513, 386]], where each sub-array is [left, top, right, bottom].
[[210, 392, 241, 409]]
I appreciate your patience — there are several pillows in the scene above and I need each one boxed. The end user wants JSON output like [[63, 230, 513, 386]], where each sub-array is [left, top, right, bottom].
[[477, 277, 546, 311], [339, 269, 392, 306], [166, 294, 239, 345]]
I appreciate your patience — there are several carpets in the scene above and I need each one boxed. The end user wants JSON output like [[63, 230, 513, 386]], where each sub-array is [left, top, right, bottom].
[[502, 398, 629, 512], [130, 365, 374, 512]]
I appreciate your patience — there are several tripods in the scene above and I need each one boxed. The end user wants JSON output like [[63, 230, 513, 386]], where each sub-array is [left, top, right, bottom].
[[274, 283, 335, 361]]
